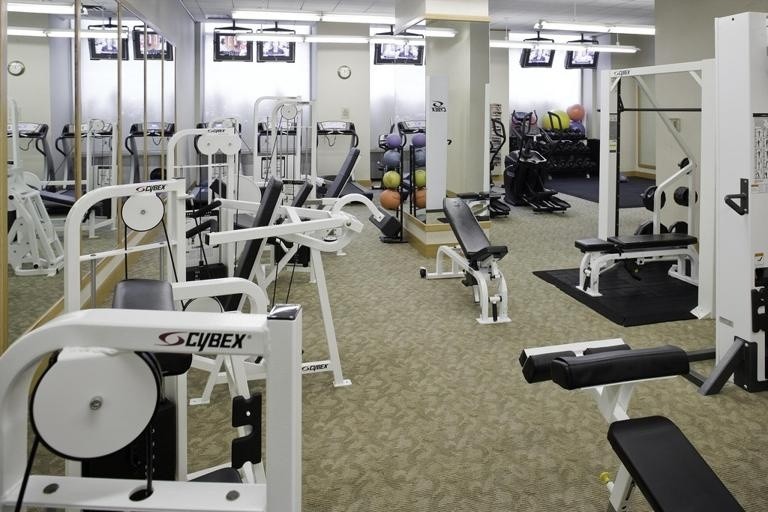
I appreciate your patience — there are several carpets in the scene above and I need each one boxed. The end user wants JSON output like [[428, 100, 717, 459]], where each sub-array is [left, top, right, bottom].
[[544, 177, 655, 208], [532, 263, 698, 327]]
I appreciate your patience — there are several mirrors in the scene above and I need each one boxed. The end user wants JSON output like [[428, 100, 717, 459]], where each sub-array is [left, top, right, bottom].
[[161, 37, 177, 202], [7, 1, 75, 346], [76, 0, 118, 293], [118, 3, 146, 242], [145, 23, 162, 192]]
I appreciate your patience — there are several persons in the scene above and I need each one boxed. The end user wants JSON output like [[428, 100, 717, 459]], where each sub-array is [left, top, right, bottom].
[[380, 43, 401, 60], [220, 36, 248, 57], [527, 47, 546, 61], [571, 50, 594, 64], [400, 46, 417, 60], [265, 42, 288, 56], [99, 39, 118, 56], [138, 33, 168, 58]]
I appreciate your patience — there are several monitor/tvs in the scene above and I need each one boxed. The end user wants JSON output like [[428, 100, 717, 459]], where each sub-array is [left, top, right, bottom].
[[565, 51, 599, 69], [374, 32, 423, 65], [214, 32, 253, 62], [132, 30, 173, 61], [520, 49, 555, 67], [88, 38, 128, 61], [257, 41, 295, 63]]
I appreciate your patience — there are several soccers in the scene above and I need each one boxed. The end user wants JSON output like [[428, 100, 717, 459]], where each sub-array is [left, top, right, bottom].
[[412, 132, 426, 208], [380, 134, 401, 208]]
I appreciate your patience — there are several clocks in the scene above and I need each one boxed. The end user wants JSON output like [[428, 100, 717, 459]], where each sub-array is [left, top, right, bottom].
[[338, 65, 351, 79]]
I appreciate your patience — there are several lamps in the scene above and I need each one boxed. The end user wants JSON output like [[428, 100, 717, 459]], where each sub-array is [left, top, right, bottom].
[[488, 17, 640, 53], [235, 22, 425, 48], [533, 1, 655, 36], [397, 26, 458, 39], [233, 10, 432, 26]]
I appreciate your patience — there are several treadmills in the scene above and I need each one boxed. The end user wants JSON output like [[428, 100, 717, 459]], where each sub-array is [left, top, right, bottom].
[[129, 125, 172, 201], [185, 122, 242, 207], [316, 120, 373, 204], [54, 123, 113, 198], [5, 123, 55, 221]]
